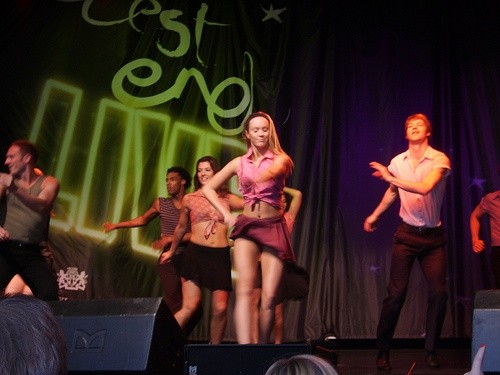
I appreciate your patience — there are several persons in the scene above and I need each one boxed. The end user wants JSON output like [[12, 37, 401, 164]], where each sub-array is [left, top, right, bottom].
[[250, 188, 301, 346], [202, 112, 295, 344], [103, 166, 205, 343], [0, 141, 66, 375], [363, 113, 451, 372], [463, 344, 486, 375], [470, 190, 500, 291], [265, 355, 338, 375], [158, 156, 246, 346]]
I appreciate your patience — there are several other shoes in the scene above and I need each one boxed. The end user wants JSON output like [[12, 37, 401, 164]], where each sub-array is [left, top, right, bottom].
[[376, 355, 392, 370], [425, 351, 440, 368]]
